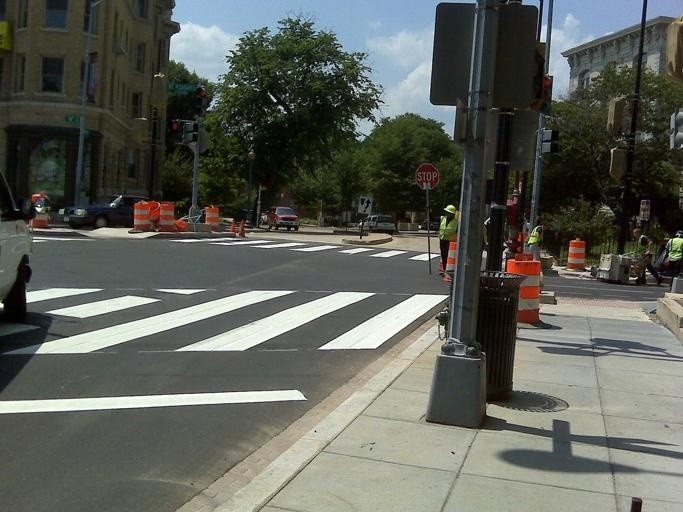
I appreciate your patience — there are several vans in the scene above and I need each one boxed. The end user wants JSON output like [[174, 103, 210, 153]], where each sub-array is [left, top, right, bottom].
[[0, 167, 36, 316]]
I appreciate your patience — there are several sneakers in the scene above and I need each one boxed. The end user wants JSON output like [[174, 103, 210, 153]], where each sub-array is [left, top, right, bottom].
[[636, 276, 664, 287]]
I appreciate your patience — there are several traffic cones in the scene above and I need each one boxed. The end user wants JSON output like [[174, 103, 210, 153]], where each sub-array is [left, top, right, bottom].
[[230, 220, 236, 236], [238, 221, 245, 237]]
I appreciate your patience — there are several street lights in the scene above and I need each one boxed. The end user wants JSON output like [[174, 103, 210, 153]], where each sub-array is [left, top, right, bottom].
[[245, 149, 255, 229]]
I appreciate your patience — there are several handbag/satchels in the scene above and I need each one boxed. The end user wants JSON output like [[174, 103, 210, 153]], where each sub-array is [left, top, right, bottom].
[[653, 248, 670, 273]]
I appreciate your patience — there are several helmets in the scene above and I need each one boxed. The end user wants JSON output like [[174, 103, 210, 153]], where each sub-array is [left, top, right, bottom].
[[443, 204, 457, 214]]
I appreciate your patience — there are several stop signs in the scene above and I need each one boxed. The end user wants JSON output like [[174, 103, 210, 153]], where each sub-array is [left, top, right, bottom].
[[415, 163, 440, 190]]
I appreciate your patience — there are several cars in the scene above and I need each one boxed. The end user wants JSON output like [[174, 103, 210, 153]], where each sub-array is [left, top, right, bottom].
[[260, 207, 299, 231], [58, 194, 150, 230], [421, 217, 440, 230], [359, 215, 396, 233]]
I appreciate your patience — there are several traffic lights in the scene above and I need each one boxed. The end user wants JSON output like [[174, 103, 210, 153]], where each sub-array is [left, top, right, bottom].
[[542, 127, 559, 154], [541, 76, 553, 111], [170, 119, 198, 142], [195, 86, 206, 116]]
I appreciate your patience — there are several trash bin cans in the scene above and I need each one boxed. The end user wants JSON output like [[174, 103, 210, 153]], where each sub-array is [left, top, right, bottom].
[[445, 270, 528, 395]]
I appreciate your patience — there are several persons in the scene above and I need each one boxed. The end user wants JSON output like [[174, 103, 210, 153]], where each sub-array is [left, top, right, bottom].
[[437, 203, 458, 277], [662, 229, 683, 291], [633, 228, 663, 287], [483, 216, 490, 252], [527, 222, 550, 262]]
